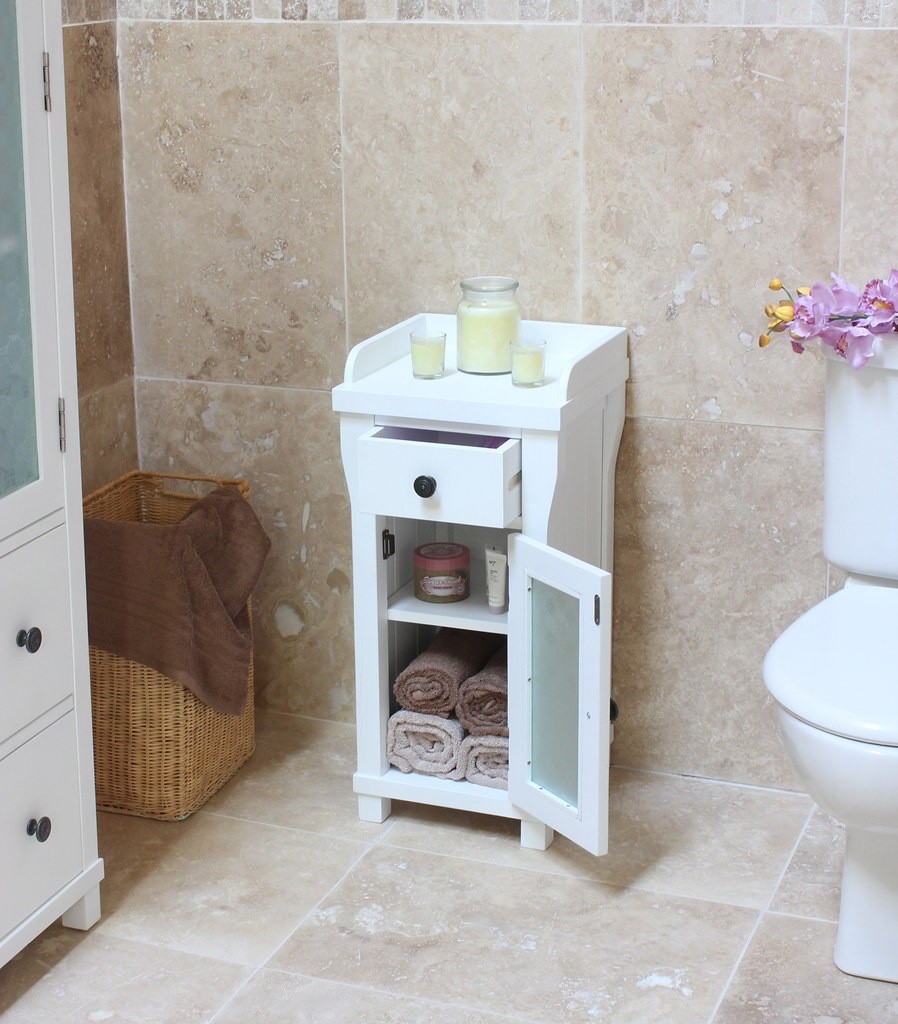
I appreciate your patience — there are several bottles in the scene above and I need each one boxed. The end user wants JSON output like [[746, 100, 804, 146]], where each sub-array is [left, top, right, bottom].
[[456, 276, 521, 375]]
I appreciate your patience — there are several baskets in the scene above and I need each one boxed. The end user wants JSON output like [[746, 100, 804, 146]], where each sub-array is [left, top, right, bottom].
[[82, 470, 255, 822]]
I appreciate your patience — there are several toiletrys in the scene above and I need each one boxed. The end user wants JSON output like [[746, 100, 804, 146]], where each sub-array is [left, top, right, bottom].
[[485, 548, 507, 614], [413, 541, 471, 603]]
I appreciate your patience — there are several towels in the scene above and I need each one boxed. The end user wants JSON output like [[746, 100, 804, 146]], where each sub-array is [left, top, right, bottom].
[[455, 644, 509, 737], [83, 484, 273, 718], [435, 735, 509, 790], [386, 710, 464, 774], [393, 630, 490, 719]]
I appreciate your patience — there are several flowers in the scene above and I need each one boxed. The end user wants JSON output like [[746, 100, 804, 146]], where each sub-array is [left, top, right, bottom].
[[755, 268, 898, 372]]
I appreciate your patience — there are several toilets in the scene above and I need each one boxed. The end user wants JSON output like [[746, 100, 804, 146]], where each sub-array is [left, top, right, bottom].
[[758, 331, 898, 990]]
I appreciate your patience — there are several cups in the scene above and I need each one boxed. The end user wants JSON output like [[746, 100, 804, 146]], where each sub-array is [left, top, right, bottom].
[[411, 329, 447, 380], [509, 338, 546, 388]]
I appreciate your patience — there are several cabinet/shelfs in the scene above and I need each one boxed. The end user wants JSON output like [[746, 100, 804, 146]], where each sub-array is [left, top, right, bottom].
[[0, 0, 103, 974], [329, 310, 633, 855]]
